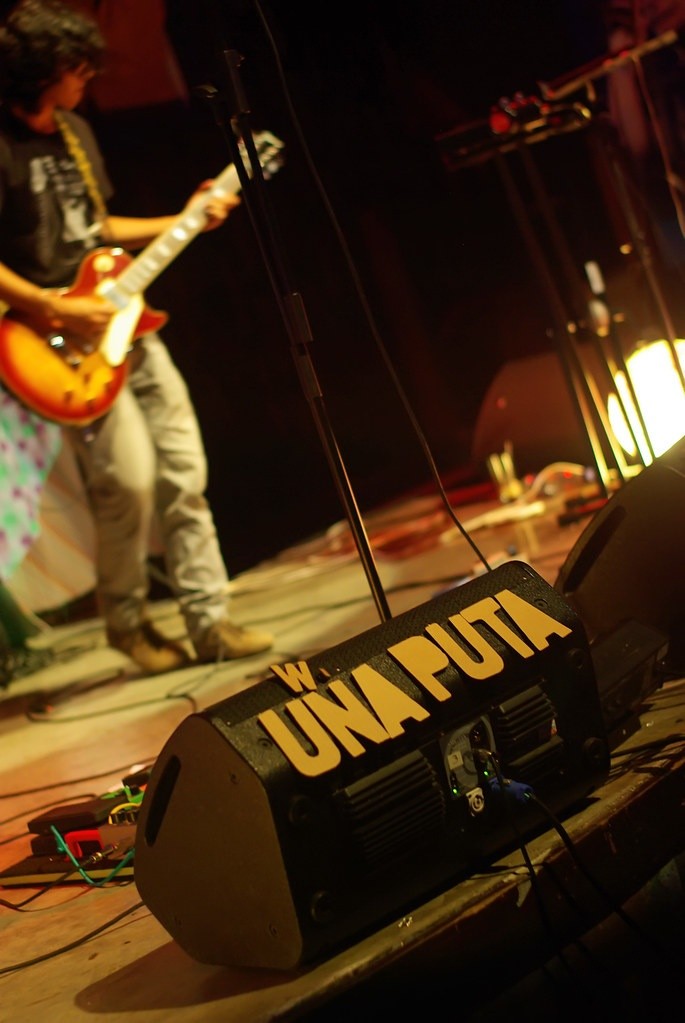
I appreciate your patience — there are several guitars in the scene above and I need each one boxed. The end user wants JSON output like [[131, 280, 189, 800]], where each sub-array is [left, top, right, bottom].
[[2, 123, 286, 427]]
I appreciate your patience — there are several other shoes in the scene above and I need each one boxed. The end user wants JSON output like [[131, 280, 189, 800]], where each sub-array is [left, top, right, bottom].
[[192, 623, 273, 661], [106, 621, 191, 671]]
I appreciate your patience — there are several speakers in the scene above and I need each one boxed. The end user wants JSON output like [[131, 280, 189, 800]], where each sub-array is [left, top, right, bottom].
[[552, 436, 685, 725], [132, 562, 611, 972], [474, 340, 663, 473]]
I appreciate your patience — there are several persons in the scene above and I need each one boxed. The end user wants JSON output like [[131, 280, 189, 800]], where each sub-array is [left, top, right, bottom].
[[0, 1, 272, 677]]
